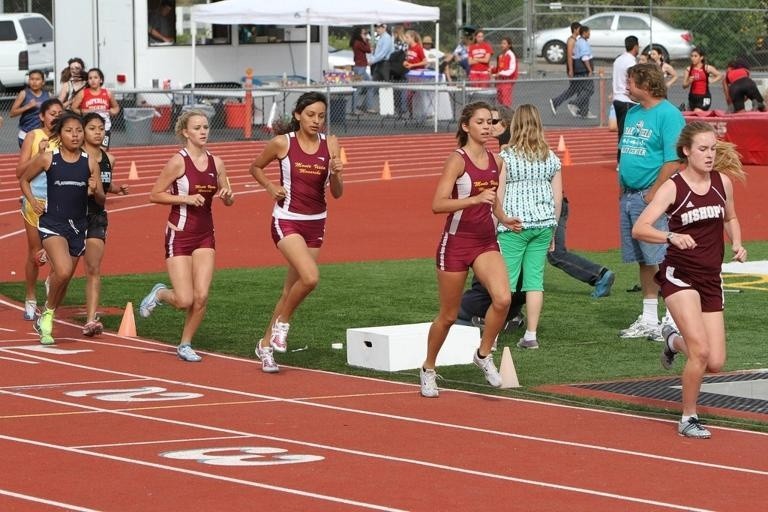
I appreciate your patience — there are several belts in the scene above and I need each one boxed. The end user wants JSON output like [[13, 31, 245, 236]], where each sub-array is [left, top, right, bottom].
[[624, 188, 647, 193]]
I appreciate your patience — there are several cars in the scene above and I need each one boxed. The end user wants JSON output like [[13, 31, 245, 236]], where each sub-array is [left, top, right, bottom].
[[328, 46, 379, 94], [527, 11, 695, 66]]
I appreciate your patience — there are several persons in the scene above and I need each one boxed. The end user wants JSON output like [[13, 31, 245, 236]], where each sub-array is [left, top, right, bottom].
[[722, 60, 767, 113], [34, 112, 129, 337], [468, 30, 495, 88], [367, 25, 394, 93], [491, 104, 563, 351], [651, 47, 678, 87], [491, 104, 616, 298], [682, 46, 724, 110], [633, 120, 748, 439], [147, 3, 175, 43], [423, 35, 454, 85], [612, 35, 640, 168], [349, 27, 377, 115], [391, 26, 408, 113], [240, 25, 255, 42], [403, 29, 427, 119], [9, 69, 55, 202], [453, 25, 474, 69], [549, 22, 583, 118], [57, 57, 87, 111], [419, 100, 524, 398], [568, 26, 597, 119], [639, 53, 655, 65], [616, 63, 687, 342], [72, 68, 121, 151], [139, 108, 235, 363], [459, 264, 527, 332], [15, 98, 65, 321], [19, 108, 106, 345], [491, 37, 518, 107], [249, 91, 344, 374]]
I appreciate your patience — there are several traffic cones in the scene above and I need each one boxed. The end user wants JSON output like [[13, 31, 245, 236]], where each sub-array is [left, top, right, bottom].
[[116, 300, 139, 338], [561, 150, 574, 167], [558, 136, 568, 152], [496, 346, 521, 390], [381, 161, 392, 180], [128, 160, 139, 181], [339, 148, 348, 165]]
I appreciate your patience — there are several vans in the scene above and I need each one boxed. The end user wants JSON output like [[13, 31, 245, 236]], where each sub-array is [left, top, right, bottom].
[[1, 13, 54, 88]]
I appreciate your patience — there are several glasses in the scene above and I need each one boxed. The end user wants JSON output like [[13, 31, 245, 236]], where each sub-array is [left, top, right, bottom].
[[492, 118, 501, 124]]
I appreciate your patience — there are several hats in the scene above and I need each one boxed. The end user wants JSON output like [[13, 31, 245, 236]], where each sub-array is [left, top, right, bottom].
[[423, 36, 433, 43]]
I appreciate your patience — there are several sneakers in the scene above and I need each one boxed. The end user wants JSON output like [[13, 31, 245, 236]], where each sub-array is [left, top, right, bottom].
[[550, 98, 557, 115], [269, 316, 290, 353], [255, 339, 279, 372], [24, 300, 37, 320], [567, 102, 578, 117], [472, 316, 485, 327], [580, 112, 597, 119], [678, 416, 711, 439], [35, 248, 47, 266], [517, 338, 538, 349], [32, 311, 54, 344], [82, 320, 103, 336], [472, 348, 502, 386], [591, 270, 615, 297], [660, 324, 679, 370], [504, 312, 524, 333], [140, 283, 166, 317], [177, 345, 201, 361], [420, 366, 443, 397], [617, 315, 661, 338]]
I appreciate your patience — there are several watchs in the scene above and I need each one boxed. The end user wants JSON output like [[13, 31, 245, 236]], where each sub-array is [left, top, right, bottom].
[[665, 231, 674, 244]]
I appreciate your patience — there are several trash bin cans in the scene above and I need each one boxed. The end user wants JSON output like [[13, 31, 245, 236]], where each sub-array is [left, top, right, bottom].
[[182, 105, 216, 126], [124, 108, 155, 145]]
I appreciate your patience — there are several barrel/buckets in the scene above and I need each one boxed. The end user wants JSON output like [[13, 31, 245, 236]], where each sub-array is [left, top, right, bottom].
[[150, 105, 171, 132], [225, 101, 250, 128]]
